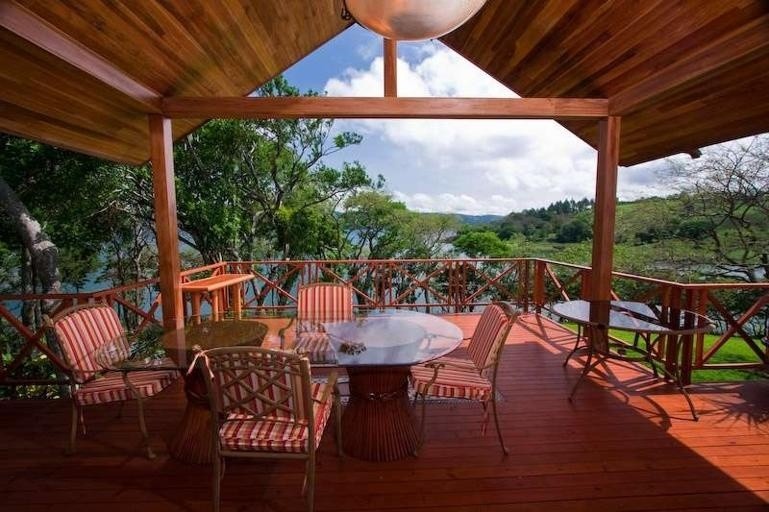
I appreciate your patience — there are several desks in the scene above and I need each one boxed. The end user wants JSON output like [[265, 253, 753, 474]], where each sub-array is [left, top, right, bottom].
[[550, 299, 718, 420], [181, 272, 256, 313], [95, 310, 464, 463]]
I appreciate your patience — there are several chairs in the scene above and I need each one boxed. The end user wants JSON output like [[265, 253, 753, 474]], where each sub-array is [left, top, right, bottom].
[[279, 282, 353, 364], [40, 299, 182, 461], [409, 301, 521, 460], [191, 344, 344, 512]]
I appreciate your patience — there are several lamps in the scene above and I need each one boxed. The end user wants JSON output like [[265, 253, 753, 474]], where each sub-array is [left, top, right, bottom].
[[339, 0, 487, 40]]
